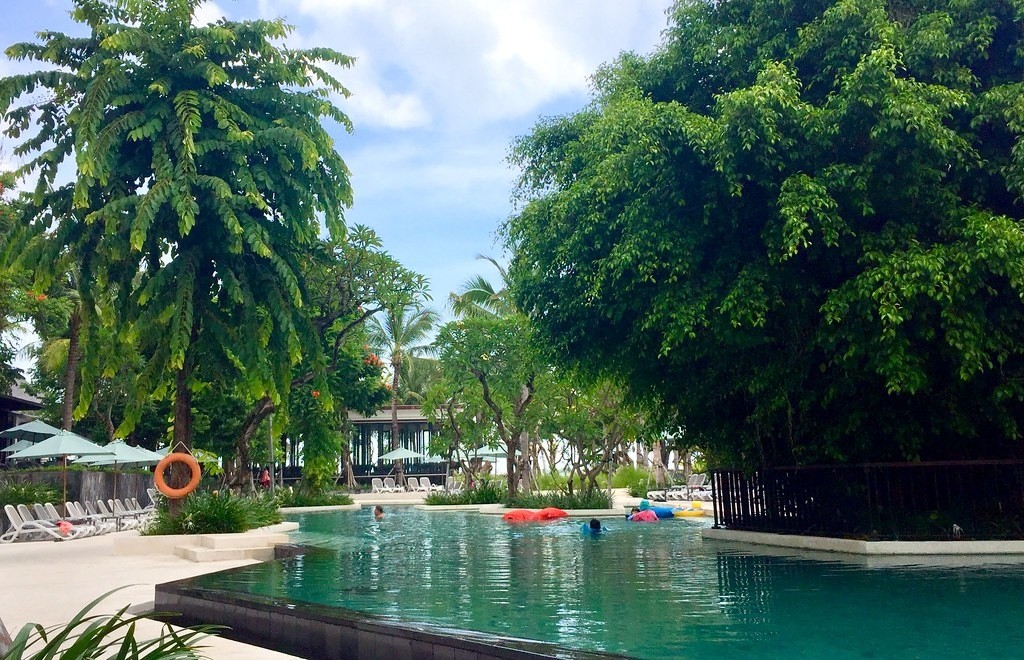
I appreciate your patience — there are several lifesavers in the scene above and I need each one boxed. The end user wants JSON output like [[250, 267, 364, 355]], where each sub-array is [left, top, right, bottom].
[[152, 451, 203, 500]]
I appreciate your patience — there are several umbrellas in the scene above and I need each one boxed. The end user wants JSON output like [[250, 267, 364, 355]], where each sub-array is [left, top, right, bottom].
[[426, 443, 522, 482], [0, 419, 220, 521], [379, 447, 425, 487]]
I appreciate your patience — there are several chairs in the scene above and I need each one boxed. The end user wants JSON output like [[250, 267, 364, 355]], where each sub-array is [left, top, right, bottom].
[[383, 478, 404, 493], [497, 480, 503, 488], [372, 478, 391, 494], [438, 480, 463, 496], [0, 488, 155, 544], [646, 473, 749, 502], [420, 477, 440, 491], [407, 478, 427, 492]]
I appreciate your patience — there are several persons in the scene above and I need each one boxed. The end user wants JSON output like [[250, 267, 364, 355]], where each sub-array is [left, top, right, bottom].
[[627, 507, 641, 520], [374, 506, 384, 520], [580, 519, 602, 535]]
